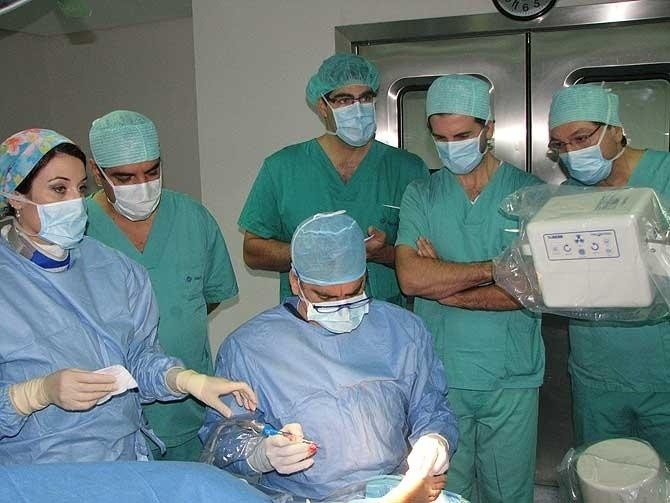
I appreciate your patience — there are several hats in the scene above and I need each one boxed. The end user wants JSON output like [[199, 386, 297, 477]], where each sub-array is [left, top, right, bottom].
[[290, 212, 367, 287], [549, 84, 623, 133], [89, 110, 161, 168], [306, 52, 382, 126], [426, 74, 493, 122], [1, 126, 77, 216]]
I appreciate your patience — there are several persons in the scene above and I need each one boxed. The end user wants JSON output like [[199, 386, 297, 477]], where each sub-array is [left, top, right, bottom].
[[546, 84, 670, 467], [0, 127, 259, 465], [198, 210, 460, 503], [376, 465, 447, 503], [83, 109, 239, 462], [238, 53, 432, 312], [393, 74, 549, 503]]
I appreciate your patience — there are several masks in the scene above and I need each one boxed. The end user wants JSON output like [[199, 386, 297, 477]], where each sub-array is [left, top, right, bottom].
[[308, 295, 369, 334], [433, 138, 485, 175], [35, 198, 88, 249], [112, 179, 163, 222], [557, 143, 615, 186], [332, 102, 376, 147]]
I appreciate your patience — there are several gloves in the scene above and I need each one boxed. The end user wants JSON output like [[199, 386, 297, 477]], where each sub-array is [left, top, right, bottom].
[[245, 423, 317, 476], [8, 367, 120, 412], [164, 366, 257, 419], [406, 433, 449, 478]]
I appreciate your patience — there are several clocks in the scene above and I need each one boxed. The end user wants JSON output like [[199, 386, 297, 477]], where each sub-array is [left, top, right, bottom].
[[493, 0, 556, 21]]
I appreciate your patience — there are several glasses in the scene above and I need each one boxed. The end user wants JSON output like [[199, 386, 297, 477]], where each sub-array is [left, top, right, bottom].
[[293, 264, 373, 313], [326, 93, 376, 107], [547, 124, 602, 153]]
